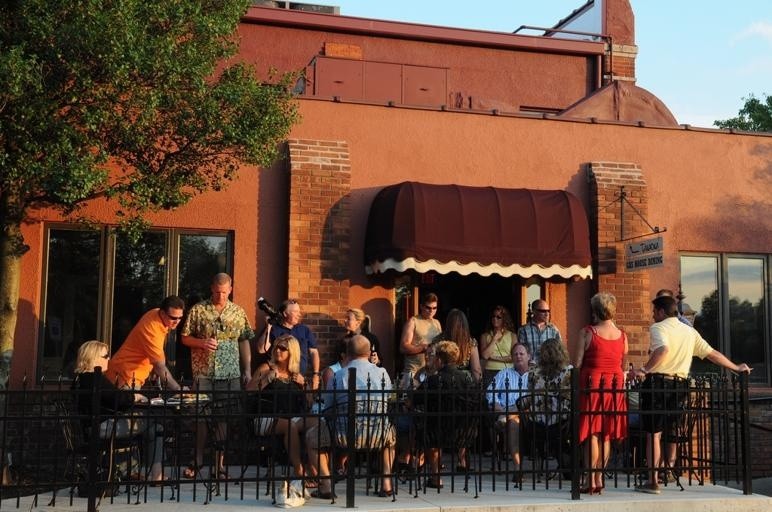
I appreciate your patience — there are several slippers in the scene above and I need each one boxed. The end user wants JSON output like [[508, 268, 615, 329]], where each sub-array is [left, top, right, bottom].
[[150, 477, 174, 487], [219, 471, 232, 480], [377, 490, 392, 496], [183, 463, 204, 478], [423, 478, 444, 488], [310, 489, 332, 499]]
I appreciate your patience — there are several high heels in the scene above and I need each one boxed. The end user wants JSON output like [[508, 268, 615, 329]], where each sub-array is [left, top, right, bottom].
[[579, 486, 598, 495], [596, 486, 605, 495]]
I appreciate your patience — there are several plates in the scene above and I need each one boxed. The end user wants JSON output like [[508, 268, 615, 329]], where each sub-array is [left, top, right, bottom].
[[169, 395, 212, 405]]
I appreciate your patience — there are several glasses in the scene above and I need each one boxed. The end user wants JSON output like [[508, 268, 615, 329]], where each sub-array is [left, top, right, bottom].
[[168, 315, 183, 320], [217, 317, 224, 332], [536, 309, 550, 312], [286, 298, 297, 304], [493, 315, 502, 319], [101, 354, 108, 358], [276, 344, 288, 351], [425, 305, 437, 309]]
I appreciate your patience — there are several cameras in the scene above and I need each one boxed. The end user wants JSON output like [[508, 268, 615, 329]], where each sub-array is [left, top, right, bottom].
[[257, 298, 283, 326]]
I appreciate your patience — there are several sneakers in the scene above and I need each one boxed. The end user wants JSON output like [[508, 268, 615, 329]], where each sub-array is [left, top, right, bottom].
[[665, 474, 677, 481], [635, 484, 660, 494], [457, 466, 474, 471], [512, 474, 524, 481]]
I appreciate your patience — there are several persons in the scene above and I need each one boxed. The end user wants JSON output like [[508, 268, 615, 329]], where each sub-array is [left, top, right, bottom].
[[573, 292, 628, 494], [322, 338, 350, 392], [180, 274, 255, 480], [655, 289, 695, 328], [480, 307, 519, 383], [413, 344, 439, 390], [255, 299, 321, 389], [103, 296, 193, 399], [397, 293, 442, 463], [624, 363, 649, 478], [486, 343, 531, 482], [433, 308, 482, 471], [518, 338, 573, 447], [246, 334, 322, 488], [344, 308, 383, 368], [517, 299, 563, 360], [410, 341, 472, 489], [627, 297, 754, 493], [71, 340, 177, 488], [304, 334, 397, 496]]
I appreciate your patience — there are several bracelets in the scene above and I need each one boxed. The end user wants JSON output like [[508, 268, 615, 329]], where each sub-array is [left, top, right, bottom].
[[640, 366, 649, 374], [312, 371, 319, 375]]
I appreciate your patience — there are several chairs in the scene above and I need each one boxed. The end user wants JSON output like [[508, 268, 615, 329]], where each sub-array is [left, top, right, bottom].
[[679, 396, 702, 490]]
